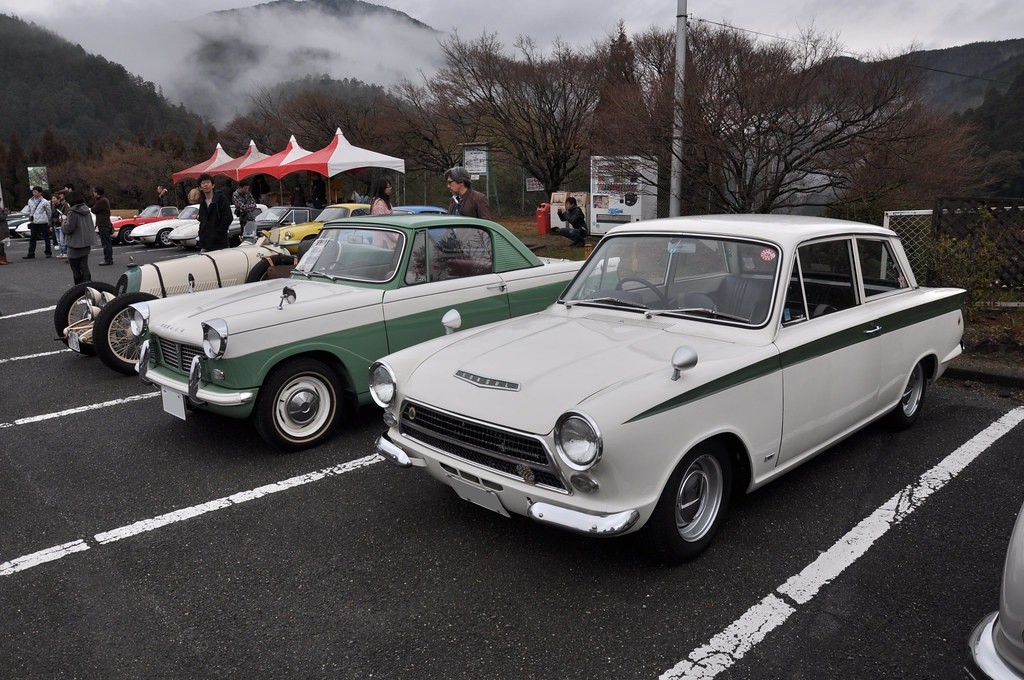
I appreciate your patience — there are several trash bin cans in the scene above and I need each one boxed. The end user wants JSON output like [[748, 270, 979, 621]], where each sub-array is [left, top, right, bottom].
[[536, 203, 551, 236]]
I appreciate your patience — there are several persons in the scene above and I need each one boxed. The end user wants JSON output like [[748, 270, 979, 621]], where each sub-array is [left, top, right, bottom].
[[91, 185, 114, 266], [370, 178, 397, 249], [22, 186, 52, 259], [50, 183, 80, 263], [59, 191, 97, 284], [444, 167, 492, 252], [557, 197, 589, 248], [158, 172, 326, 250], [0, 198, 13, 265]]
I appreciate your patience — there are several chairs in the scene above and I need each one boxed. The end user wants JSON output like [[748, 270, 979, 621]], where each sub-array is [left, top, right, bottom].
[[716, 275, 762, 321]]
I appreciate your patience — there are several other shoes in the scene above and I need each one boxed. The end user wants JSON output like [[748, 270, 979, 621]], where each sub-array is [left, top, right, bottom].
[[46, 254, 51, 258], [22, 256, 35, 259], [53, 245, 60, 251], [98, 260, 114, 265], [56, 253, 68, 259], [572, 239, 585, 247]]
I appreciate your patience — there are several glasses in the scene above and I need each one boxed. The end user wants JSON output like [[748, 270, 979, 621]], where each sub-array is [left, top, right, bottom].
[[386, 186, 393, 190], [446, 176, 461, 185]]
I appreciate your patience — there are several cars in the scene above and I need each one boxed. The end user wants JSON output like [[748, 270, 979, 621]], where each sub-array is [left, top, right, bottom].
[[127, 205, 201, 245], [260, 203, 372, 260], [127, 216, 620, 451], [332, 205, 451, 250], [369, 212, 967, 560], [6, 209, 120, 239], [230, 206, 325, 243], [168, 205, 278, 248], [95, 205, 199, 245]]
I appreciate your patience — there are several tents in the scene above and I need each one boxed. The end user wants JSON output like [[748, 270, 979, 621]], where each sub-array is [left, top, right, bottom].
[[172, 127, 407, 207]]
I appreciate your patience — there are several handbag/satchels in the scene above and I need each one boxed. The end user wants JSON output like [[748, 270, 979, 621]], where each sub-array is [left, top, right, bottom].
[[29, 216, 33, 222], [110, 223, 114, 234], [247, 208, 262, 221]]
